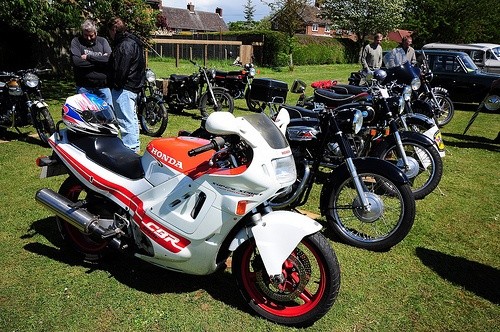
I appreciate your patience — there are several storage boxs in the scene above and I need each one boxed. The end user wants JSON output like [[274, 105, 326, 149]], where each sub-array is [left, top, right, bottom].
[[250, 78, 289, 102], [156, 79, 169, 96]]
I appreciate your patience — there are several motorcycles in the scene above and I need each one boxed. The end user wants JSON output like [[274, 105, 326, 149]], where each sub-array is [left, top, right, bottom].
[[35, 89, 343, 328], [208, 51, 267, 112], [161, 60, 234, 119], [1, 69, 56, 144], [250, 50, 455, 252], [139, 68, 169, 137]]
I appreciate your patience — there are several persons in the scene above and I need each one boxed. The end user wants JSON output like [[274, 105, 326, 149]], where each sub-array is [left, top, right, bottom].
[[70, 18, 114, 110], [360, 33, 383, 75], [389, 35, 417, 68], [107, 16, 144, 153]]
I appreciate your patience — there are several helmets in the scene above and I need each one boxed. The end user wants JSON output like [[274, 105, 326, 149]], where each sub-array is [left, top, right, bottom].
[[61, 92, 120, 136]]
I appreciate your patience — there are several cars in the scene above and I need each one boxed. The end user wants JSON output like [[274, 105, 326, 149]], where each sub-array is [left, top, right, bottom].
[[382, 43, 500, 113]]
